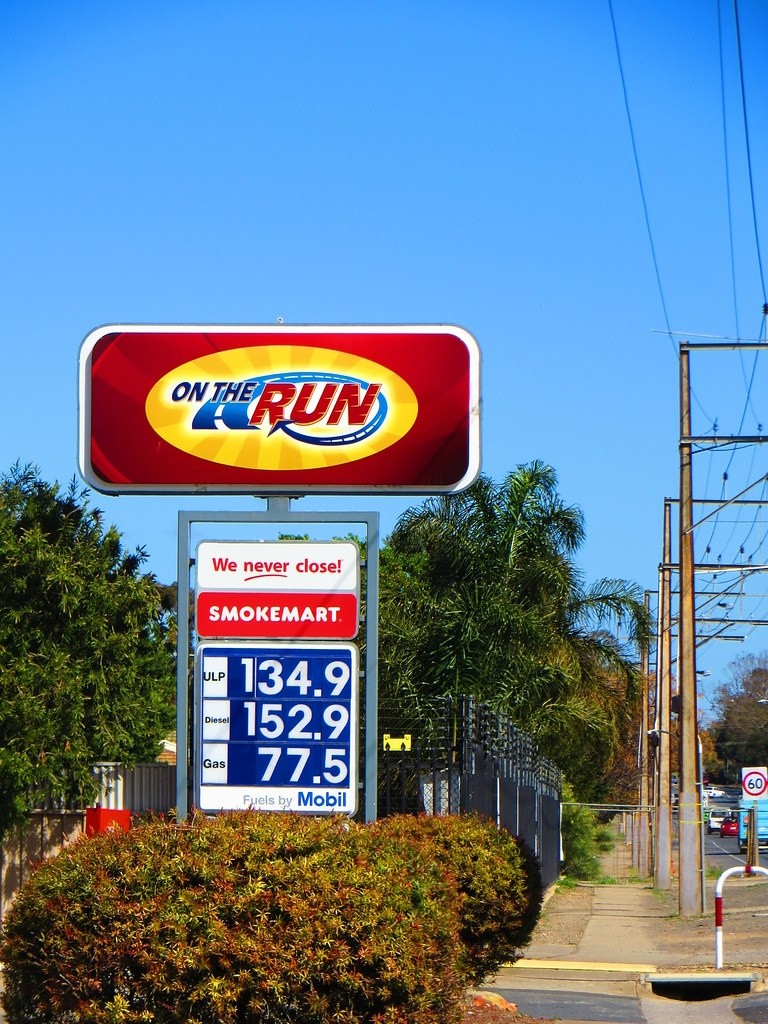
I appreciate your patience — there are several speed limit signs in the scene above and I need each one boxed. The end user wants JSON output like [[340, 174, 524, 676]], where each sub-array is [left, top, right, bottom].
[[741, 765, 767, 801]]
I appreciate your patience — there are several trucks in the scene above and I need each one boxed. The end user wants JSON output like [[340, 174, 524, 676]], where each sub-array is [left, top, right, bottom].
[[737, 794, 768, 854]]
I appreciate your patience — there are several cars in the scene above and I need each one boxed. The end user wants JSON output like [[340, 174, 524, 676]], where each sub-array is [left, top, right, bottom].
[[668, 786, 740, 838]]
